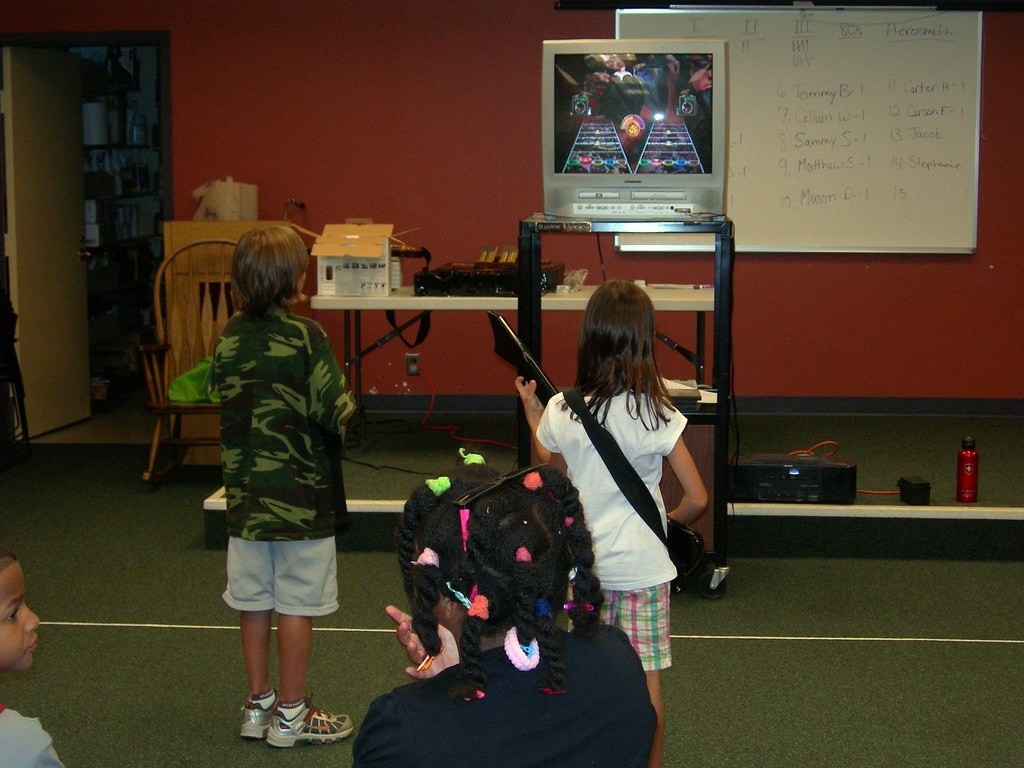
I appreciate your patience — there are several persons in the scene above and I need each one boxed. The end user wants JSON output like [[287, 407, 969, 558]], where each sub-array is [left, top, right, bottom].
[[0, 547, 64, 768], [514, 279, 708, 768], [348, 448, 658, 768], [214, 224, 356, 747]]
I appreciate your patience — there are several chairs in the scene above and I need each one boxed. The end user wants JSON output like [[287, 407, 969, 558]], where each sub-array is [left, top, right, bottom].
[[134, 237, 244, 487]]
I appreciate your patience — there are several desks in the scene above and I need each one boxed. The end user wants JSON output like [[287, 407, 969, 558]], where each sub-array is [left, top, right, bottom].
[[310, 281, 717, 441]]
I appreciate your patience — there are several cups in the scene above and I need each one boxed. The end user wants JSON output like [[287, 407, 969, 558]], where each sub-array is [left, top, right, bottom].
[[128, 114, 146, 145]]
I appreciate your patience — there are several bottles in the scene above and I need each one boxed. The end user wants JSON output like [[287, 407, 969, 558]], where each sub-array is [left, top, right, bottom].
[[956, 436, 978, 503]]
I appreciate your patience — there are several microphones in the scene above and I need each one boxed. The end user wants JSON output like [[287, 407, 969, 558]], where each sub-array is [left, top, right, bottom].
[[289, 198, 305, 208]]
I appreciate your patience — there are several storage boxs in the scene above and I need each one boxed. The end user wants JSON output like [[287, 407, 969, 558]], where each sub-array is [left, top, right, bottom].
[[308, 220, 397, 298]]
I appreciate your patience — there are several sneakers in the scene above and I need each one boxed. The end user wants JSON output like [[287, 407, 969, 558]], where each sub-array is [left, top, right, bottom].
[[266, 688, 353, 747], [240, 688, 279, 738]]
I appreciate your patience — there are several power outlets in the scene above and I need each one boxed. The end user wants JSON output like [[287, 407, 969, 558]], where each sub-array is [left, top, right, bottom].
[[406, 353, 420, 376]]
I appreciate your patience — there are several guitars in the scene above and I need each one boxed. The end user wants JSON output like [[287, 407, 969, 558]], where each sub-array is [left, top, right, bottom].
[[489, 308, 704, 587]]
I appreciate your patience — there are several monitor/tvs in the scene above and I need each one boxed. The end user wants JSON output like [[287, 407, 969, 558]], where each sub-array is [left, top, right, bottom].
[[541, 37, 729, 222]]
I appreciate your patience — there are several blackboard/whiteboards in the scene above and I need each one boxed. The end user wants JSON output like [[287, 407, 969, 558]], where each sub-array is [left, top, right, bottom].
[[613, 7, 983, 254]]
